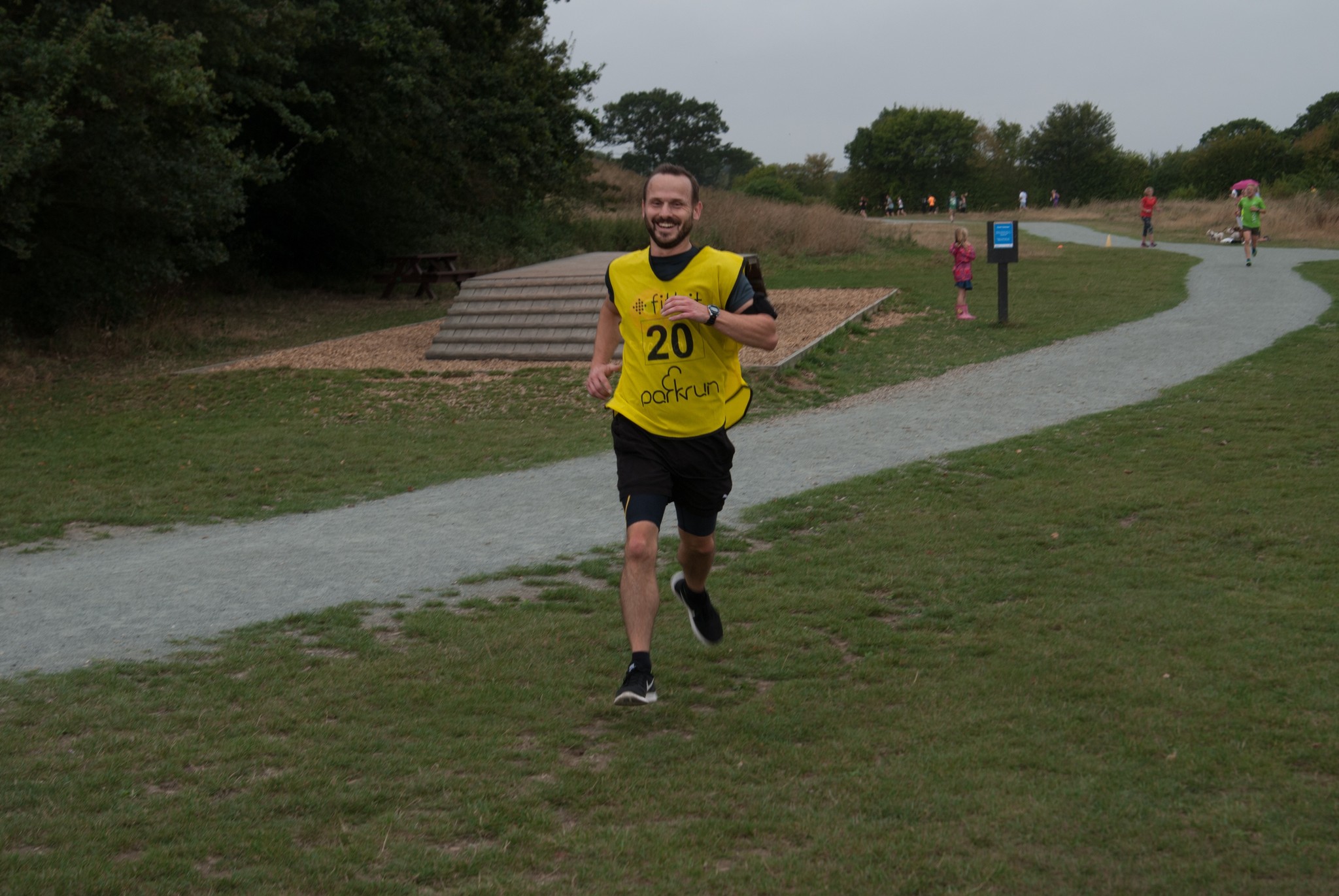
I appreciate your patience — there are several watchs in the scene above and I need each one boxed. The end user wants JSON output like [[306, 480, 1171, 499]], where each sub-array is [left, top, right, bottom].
[[703, 304, 720, 326], [1257, 208, 1260, 212]]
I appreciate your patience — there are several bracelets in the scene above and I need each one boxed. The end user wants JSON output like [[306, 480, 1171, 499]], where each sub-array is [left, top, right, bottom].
[[1146, 209, 1149, 212]]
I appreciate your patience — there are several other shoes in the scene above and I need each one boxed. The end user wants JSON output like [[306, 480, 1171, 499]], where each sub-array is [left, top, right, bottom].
[[1150, 242, 1157, 247], [1247, 260, 1251, 267], [1142, 243, 1150, 247], [1252, 247, 1258, 257]]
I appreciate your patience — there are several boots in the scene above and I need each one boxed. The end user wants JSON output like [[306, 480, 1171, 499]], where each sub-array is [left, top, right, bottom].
[[955, 305, 976, 319]]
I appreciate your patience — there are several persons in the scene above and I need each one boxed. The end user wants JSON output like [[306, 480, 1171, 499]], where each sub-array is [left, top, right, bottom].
[[586, 162, 777, 706], [858, 183, 1319, 320]]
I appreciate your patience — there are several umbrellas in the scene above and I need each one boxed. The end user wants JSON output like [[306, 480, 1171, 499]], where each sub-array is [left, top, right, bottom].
[[1230, 179, 1260, 191]]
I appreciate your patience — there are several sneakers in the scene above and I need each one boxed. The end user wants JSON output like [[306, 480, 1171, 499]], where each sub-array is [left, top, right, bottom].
[[671, 570, 723, 646], [613, 662, 658, 705]]
